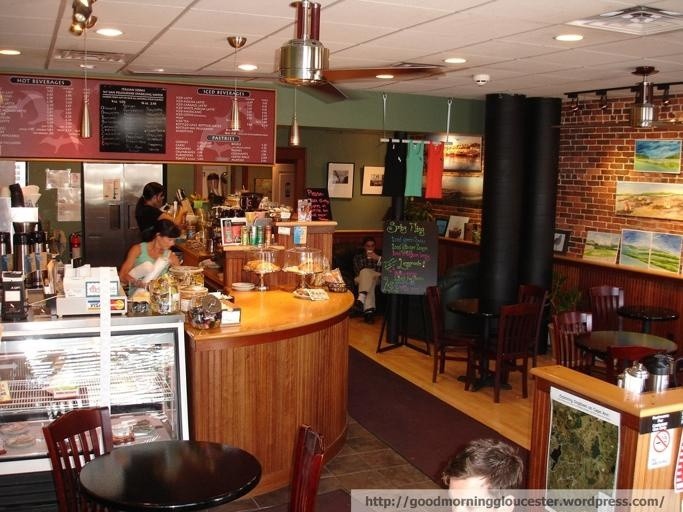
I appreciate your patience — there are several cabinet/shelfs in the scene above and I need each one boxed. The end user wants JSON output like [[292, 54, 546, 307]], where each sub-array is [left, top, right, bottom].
[[0, 306, 192, 511]]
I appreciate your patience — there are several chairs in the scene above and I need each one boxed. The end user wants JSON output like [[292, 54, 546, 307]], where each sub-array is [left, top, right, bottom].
[[40, 404, 117, 512], [337, 244, 681, 408], [285, 422, 330, 512]]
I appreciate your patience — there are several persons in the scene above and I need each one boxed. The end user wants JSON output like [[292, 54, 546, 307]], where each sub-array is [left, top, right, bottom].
[[440, 435, 524, 512], [134, 181, 165, 241], [119, 218, 180, 295], [348, 236, 383, 325]]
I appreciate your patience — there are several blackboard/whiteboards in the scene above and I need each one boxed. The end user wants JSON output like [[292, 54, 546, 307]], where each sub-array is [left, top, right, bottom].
[[305, 187, 332, 221], [379, 217, 438, 295]]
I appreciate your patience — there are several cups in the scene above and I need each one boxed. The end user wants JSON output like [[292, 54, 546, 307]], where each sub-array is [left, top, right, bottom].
[[366, 250, 373, 259]]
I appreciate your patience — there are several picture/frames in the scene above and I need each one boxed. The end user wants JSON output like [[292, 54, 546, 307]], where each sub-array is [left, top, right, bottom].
[[552, 229, 571, 256], [359, 163, 387, 197], [409, 133, 486, 178], [434, 217, 448, 238], [324, 161, 355, 201]]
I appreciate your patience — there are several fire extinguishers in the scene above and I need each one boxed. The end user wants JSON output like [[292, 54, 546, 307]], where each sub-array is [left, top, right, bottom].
[[69, 231, 83, 259]]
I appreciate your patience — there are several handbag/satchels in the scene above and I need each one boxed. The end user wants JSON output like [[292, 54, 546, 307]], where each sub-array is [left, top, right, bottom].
[[374, 256, 382, 272]]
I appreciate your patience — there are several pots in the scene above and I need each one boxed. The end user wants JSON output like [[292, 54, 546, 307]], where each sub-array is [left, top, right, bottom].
[[240, 192, 263, 208]]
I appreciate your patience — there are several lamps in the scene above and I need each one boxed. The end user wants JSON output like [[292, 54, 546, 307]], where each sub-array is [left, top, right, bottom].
[[77, 13, 99, 141], [288, 82, 301, 148], [566, 82, 671, 113], [67, 1, 97, 37], [226, 34, 247, 132]]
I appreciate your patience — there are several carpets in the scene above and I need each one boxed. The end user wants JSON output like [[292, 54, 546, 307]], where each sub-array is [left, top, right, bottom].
[[346, 308, 607, 512]]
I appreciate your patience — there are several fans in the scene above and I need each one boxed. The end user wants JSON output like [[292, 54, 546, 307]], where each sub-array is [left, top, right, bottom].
[[120, 1, 442, 105], [552, 64, 683, 133]]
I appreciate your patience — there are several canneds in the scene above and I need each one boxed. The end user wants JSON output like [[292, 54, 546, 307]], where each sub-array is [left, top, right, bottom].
[[249, 226, 257, 245], [257, 226, 264, 244], [264, 226, 272, 243], [241, 226, 249, 245]]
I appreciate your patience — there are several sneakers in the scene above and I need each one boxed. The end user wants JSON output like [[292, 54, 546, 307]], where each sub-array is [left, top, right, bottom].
[[349, 298, 365, 313], [363, 311, 376, 325]]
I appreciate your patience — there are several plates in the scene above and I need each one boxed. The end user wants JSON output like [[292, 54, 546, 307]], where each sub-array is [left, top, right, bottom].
[[231, 282, 255, 292], [0, 424, 36, 449]]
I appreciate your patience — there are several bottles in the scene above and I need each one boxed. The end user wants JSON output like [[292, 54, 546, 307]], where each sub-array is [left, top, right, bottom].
[[240, 225, 273, 246], [617, 353, 676, 392]]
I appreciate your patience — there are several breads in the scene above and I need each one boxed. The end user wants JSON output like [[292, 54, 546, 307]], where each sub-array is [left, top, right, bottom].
[[282, 260, 344, 286], [245, 260, 282, 271]]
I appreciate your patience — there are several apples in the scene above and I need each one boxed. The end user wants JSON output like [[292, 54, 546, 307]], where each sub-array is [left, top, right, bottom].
[[114, 300, 124, 310]]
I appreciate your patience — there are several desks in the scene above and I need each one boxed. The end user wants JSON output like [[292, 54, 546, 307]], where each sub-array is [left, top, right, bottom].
[[76, 440, 263, 512]]
